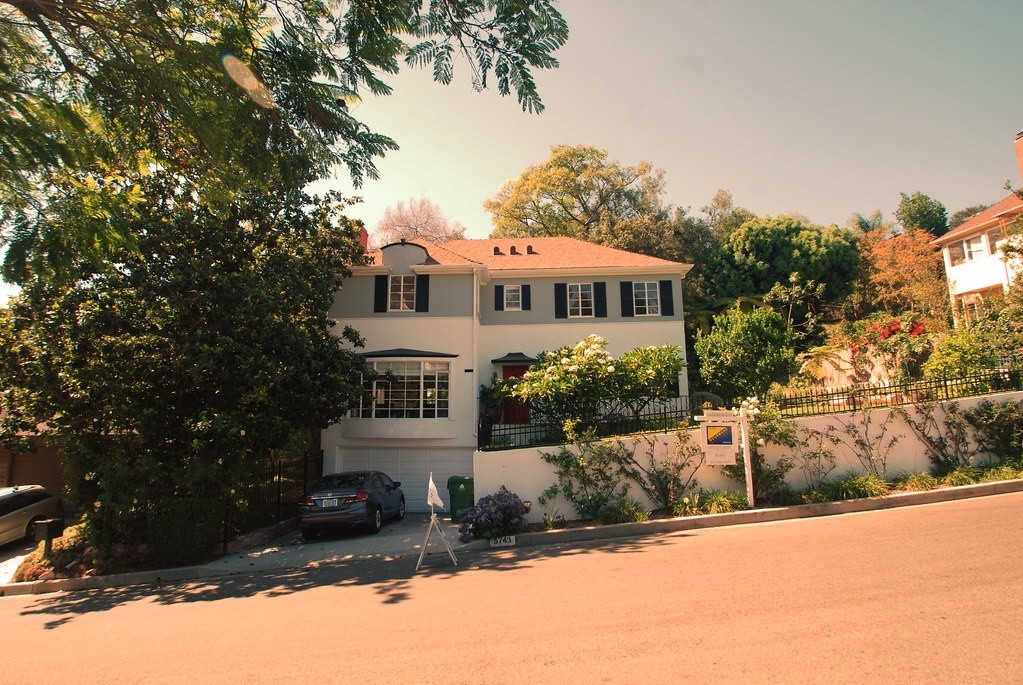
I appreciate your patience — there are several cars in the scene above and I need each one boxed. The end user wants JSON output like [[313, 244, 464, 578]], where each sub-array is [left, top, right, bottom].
[[0, 485, 66, 546], [298, 470, 405, 540]]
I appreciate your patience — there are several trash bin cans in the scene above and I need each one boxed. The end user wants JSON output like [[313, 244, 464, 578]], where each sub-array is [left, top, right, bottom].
[[447, 475, 474, 523]]
